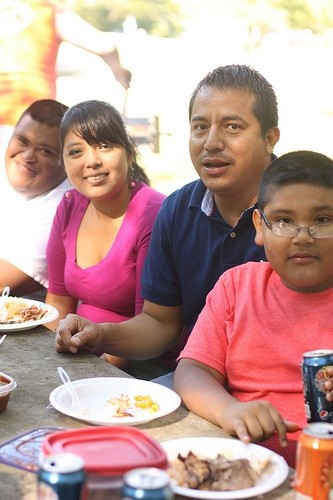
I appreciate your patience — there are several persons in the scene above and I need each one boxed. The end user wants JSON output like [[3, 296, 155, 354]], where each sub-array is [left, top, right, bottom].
[[0, 99, 76, 306], [56, 67, 280, 389], [42, 101, 188, 381], [175, 150, 333, 467]]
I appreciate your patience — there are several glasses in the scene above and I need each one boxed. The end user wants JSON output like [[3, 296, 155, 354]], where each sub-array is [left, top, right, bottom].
[[258, 209, 333, 238]]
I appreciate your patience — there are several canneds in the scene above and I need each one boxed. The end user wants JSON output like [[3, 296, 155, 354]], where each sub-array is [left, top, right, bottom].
[[295, 422, 333, 500], [299, 349, 333, 423], [119, 466, 175, 500], [36, 452, 87, 500]]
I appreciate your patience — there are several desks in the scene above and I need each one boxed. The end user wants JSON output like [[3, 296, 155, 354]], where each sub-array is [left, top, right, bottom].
[[0, 323, 296, 500]]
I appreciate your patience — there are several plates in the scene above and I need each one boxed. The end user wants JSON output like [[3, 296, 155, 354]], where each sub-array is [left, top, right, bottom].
[[160, 437, 289, 500], [0, 297, 60, 333], [49, 377, 183, 427]]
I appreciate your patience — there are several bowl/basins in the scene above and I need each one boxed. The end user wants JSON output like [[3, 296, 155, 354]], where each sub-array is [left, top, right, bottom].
[[0, 372, 17, 414], [40, 426, 169, 500]]
